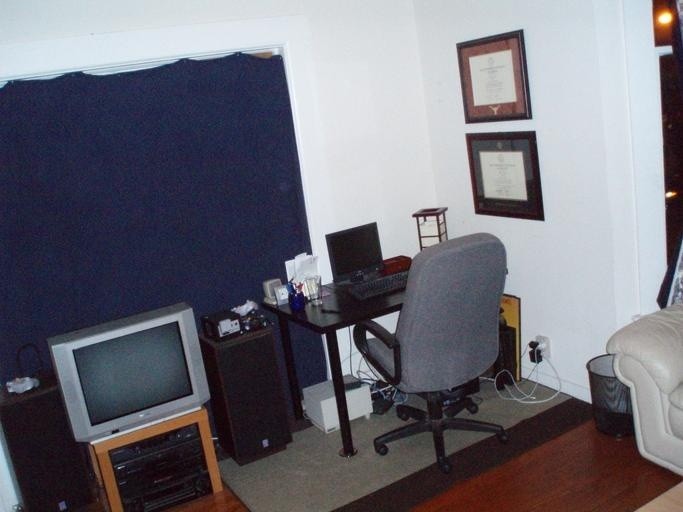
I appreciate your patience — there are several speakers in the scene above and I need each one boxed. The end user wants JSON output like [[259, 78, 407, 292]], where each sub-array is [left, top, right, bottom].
[[198, 328, 293, 465], [262, 278, 283, 306], [0, 380, 97, 511]]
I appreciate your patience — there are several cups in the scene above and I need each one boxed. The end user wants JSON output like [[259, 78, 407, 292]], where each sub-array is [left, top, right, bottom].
[[306, 275, 322, 305], [309, 306, 323, 316]]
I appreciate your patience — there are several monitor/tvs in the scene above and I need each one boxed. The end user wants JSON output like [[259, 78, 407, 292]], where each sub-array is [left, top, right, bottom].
[[325, 221, 384, 286], [47, 302, 210, 445]]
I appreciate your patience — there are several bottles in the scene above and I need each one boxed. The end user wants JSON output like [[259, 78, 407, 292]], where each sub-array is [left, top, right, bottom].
[[289, 291, 305, 309], [245, 308, 266, 333], [292, 310, 306, 320]]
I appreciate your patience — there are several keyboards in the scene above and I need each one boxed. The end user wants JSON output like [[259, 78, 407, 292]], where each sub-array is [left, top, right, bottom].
[[342, 270, 409, 301]]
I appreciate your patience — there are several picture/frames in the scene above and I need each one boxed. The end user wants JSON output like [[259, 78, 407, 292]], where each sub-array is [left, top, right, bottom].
[[455, 27, 544, 224]]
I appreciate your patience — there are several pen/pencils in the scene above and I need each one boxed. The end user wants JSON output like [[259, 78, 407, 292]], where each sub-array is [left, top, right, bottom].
[[321, 310, 340, 313]]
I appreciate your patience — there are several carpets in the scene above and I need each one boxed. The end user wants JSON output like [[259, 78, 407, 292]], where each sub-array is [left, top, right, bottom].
[[213, 378, 594, 512], [629, 480, 682, 512]]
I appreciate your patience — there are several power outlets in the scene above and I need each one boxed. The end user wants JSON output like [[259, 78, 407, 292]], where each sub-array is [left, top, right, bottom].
[[532, 335, 550, 360]]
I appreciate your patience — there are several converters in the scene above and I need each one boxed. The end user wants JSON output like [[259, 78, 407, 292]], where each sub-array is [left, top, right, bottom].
[[529, 341, 547, 363]]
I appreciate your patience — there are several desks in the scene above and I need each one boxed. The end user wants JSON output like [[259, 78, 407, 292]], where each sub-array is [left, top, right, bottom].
[[263, 270, 410, 456]]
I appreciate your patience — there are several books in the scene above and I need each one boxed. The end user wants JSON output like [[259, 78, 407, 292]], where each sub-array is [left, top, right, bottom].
[[285, 253, 321, 300]]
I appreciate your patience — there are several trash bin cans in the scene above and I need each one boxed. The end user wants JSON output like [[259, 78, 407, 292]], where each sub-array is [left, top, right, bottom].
[[587, 355, 635, 438]]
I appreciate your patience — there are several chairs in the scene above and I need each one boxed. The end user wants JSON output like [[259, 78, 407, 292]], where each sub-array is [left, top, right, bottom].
[[352, 232, 510, 473], [606, 303, 683, 475]]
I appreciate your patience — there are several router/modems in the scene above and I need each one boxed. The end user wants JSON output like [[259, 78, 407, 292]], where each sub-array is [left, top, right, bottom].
[[343, 374, 361, 391]]
